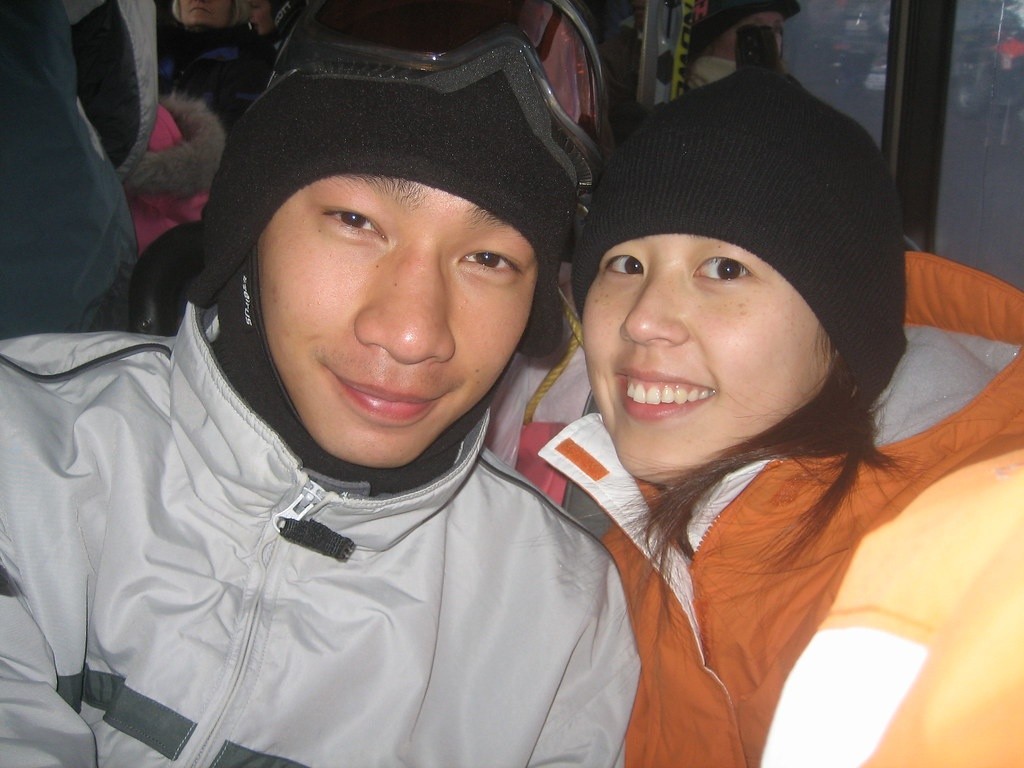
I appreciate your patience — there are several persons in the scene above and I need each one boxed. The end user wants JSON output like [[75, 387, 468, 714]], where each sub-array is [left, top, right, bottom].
[[536, 25, 1024, 768], [0, 1, 1024, 768], [0, 0, 640, 768]]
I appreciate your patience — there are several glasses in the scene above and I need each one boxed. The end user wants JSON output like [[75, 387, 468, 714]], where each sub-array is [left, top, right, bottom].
[[233, 0, 610, 243]]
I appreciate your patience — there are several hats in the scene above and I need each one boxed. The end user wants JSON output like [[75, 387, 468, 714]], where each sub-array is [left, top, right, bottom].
[[570, 60, 908, 414], [188, 0, 594, 362]]
